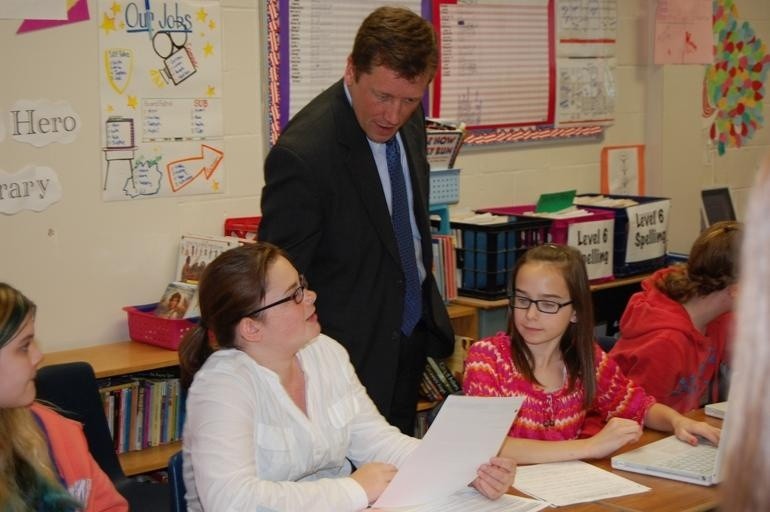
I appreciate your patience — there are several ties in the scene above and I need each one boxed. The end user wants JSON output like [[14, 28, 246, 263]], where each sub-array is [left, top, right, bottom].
[[385, 133, 423, 339]]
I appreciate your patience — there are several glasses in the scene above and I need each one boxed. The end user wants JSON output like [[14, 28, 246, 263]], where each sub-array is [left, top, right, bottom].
[[508, 294, 574, 314], [245, 274, 309, 316]]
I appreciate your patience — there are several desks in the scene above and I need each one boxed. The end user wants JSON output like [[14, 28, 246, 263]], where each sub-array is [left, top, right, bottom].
[[373, 401, 724, 512], [453, 272, 652, 313]]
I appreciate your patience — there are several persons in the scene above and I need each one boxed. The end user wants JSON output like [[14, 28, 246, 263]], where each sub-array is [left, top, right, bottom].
[[462, 241, 723, 467], [0, 282, 131, 512], [579, 218, 746, 442], [255, 4, 441, 439], [176, 242, 518, 512]]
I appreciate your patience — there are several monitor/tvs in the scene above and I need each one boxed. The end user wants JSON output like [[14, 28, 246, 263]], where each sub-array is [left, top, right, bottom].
[[701, 187, 741, 228]]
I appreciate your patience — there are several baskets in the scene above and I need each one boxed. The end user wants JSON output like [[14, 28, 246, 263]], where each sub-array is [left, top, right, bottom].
[[224, 215, 263, 243], [572, 192, 672, 280], [430, 210, 552, 302], [122, 301, 203, 351], [428, 167, 462, 206], [479, 205, 617, 285]]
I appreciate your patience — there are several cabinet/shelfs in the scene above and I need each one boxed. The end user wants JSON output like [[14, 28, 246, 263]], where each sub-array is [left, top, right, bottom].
[[38, 302, 479, 484]]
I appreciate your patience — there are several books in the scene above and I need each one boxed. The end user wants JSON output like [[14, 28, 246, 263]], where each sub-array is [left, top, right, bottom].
[[144, 233, 245, 349], [419, 116, 466, 404], [95, 367, 192, 454]]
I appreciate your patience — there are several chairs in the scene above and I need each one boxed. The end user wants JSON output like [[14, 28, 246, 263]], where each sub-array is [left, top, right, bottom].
[[32, 362, 171, 512], [168, 453, 190, 511]]
